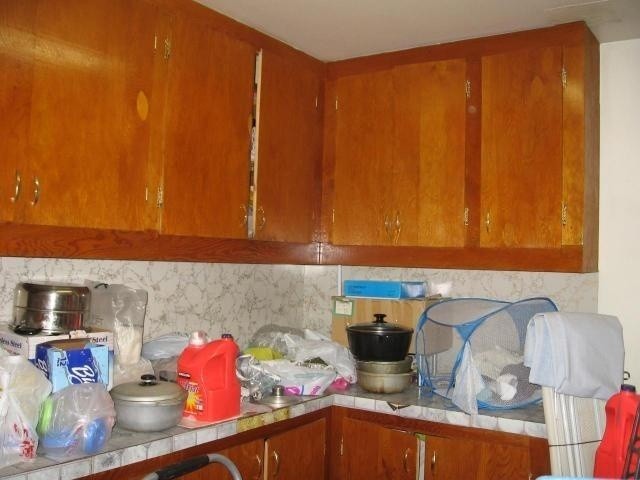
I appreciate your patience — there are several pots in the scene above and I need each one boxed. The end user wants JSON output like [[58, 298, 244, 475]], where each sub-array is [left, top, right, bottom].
[[8, 282, 93, 336], [345, 313, 415, 361], [108, 375, 188, 432]]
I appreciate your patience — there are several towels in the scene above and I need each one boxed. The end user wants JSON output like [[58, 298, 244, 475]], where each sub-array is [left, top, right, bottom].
[[522, 310, 625, 404]]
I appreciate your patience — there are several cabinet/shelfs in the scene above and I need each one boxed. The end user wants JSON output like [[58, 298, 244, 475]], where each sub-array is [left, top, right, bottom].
[[323, 35, 476, 274], [0, 0, 156, 262], [157, 0, 322, 266], [71, 404, 331, 480], [330, 393, 553, 480], [474, 17, 605, 274]]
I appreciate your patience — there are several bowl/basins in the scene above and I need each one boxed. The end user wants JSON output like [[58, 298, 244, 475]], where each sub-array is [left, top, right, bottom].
[[355, 355, 412, 373], [355, 369, 415, 393]]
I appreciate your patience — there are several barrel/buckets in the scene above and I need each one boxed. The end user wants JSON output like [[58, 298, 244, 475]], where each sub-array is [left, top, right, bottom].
[[593, 385, 640, 480], [176, 330, 242, 423]]
